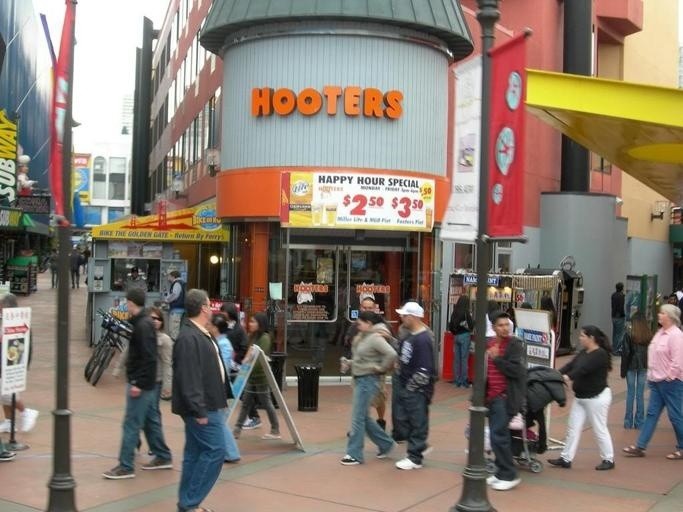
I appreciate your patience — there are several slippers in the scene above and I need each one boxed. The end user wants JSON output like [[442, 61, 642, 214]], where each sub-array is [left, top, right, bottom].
[[177, 507, 213, 512]]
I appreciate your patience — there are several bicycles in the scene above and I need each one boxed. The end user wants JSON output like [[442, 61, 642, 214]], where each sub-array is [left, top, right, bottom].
[[91, 311, 131, 385], [82, 308, 133, 380]]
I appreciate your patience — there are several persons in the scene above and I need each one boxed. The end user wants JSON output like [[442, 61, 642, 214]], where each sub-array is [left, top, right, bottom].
[[669, 294, 678, 305], [125, 267, 148, 293], [373, 300, 438, 442], [171, 289, 234, 512], [49, 249, 59, 289], [506, 307, 517, 332], [623, 303, 682, 459], [165, 271, 187, 342], [81, 246, 91, 274], [540, 291, 555, 311], [546, 326, 615, 471], [449, 296, 474, 387], [102, 288, 173, 480], [621, 312, 652, 429], [345, 297, 393, 432], [208, 314, 240, 463], [394, 302, 435, 471], [70, 250, 82, 289], [484, 312, 527, 491], [113, 306, 173, 455], [1, 292, 39, 432], [485, 299, 499, 347], [611, 282, 625, 356], [339, 312, 398, 466], [674, 281, 683, 301], [232, 312, 281, 439], [221, 302, 262, 430], [1, 440, 14, 461]]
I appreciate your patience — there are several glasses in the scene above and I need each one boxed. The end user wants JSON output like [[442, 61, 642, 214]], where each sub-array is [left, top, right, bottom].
[[151, 316, 163, 322]]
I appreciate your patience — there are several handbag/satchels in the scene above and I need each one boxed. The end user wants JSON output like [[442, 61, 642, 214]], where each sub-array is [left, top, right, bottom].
[[460, 311, 473, 332]]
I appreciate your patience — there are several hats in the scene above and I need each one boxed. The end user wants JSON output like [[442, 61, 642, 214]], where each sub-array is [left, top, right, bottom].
[[131, 267, 138, 273], [492, 311, 511, 320], [395, 302, 425, 318]]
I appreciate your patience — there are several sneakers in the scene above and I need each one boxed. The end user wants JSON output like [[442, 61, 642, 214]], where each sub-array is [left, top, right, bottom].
[[339, 455, 360, 465], [240, 416, 262, 429], [262, 432, 280, 440], [21, 409, 39, 432], [377, 453, 386, 458], [421, 445, 433, 455], [143, 459, 173, 469], [486, 475, 499, 485], [396, 458, 422, 470], [231, 431, 241, 439], [101, 466, 135, 479], [491, 478, 520, 490], [0, 419, 18, 433]]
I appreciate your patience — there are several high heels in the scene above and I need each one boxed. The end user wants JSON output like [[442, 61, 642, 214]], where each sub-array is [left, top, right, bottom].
[[148, 449, 156, 455], [136, 439, 141, 452]]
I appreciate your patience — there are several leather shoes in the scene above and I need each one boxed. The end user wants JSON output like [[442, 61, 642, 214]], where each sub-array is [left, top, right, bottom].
[[596, 460, 614, 470], [224, 458, 241, 464], [547, 458, 571, 467]]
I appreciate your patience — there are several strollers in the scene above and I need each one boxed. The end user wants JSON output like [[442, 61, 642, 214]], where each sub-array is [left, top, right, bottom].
[[464, 366, 567, 473]]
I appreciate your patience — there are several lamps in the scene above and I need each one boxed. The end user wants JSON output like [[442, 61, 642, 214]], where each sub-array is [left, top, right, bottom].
[[205, 147, 219, 177], [651, 200, 667, 219], [171, 176, 184, 199], [145, 203, 153, 214]]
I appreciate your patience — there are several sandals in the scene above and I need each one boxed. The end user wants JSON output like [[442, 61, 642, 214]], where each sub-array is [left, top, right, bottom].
[[666, 450, 683, 459], [623, 445, 645, 456]]
